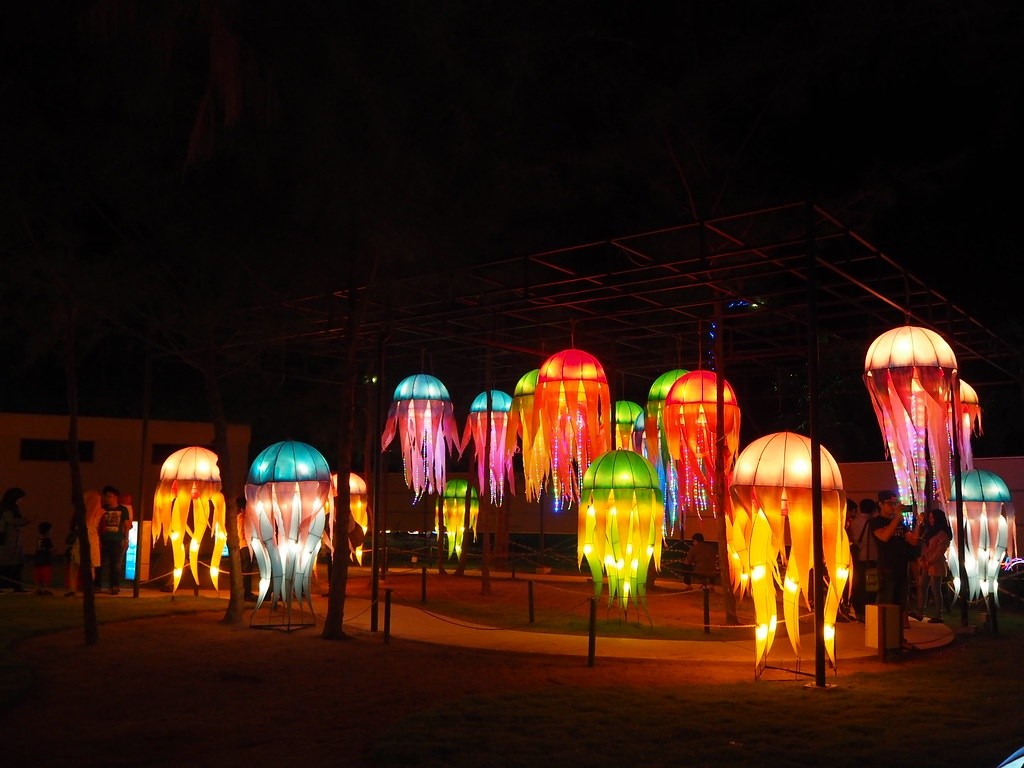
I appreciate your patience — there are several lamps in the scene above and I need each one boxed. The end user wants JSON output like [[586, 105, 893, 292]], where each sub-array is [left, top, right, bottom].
[[633, 335, 692, 549], [663, 322, 741, 527], [322, 471, 368, 570], [378, 342, 461, 505], [615, 370, 643, 451], [861, 277, 968, 521], [433, 465, 481, 562], [242, 438, 336, 633], [531, 321, 612, 512], [457, 368, 522, 509], [937, 469, 1018, 619], [504, 342, 562, 503], [577, 449, 669, 633], [724, 358, 855, 683], [946, 379, 984, 478], [152, 445, 227, 594]]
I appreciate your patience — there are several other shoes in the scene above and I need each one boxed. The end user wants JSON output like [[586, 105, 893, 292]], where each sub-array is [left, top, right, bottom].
[[43, 590, 53, 595], [263, 594, 271, 600], [110, 586, 120, 595], [908, 609, 923, 621], [928, 615, 944, 623], [13, 587, 30, 593], [64, 591, 75, 597], [244, 590, 259, 603], [91, 584, 102, 593]]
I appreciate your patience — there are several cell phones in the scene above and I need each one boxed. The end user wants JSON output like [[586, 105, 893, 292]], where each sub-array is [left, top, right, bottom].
[[902, 506, 912, 512]]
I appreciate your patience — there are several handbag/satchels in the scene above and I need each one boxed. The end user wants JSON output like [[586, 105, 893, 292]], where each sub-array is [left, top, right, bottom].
[[852, 544, 860, 560], [865, 566, 880, 592], [0, 532, 6, 544]]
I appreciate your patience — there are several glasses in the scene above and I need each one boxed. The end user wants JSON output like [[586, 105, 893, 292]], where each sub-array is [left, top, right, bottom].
[[884, 501, 899, 506]]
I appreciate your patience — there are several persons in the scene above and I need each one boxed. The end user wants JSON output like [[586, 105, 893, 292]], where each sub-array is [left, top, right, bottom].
[[869, 490, 924, 663], [682, 533, 717, 591], [33, 522, 54, 595], [321, 513, 355, 596], [63, 485, 132, 597], [0, 488, 35, 595], [235, 495, 259, 602], [824, 497, 953, 627]]
